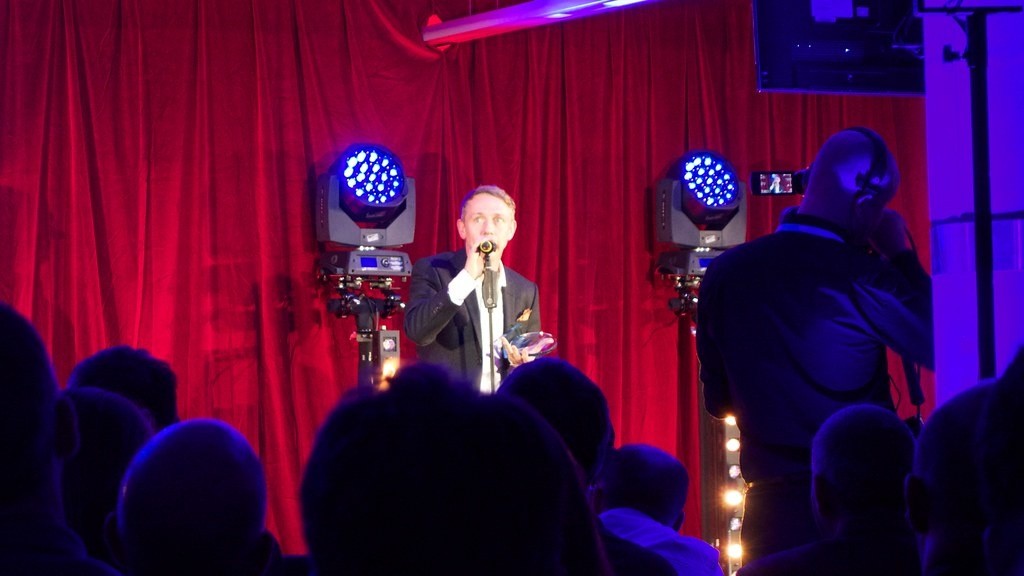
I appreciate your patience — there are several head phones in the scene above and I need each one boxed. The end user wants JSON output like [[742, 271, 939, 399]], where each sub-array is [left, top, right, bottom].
[[802, 127, 887, 220]]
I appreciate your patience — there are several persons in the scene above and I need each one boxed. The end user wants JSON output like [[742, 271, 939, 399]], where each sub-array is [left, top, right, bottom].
[[402, 184, 542, 392], [0, 304, 1024, 576], [697, 125, 935, 565]]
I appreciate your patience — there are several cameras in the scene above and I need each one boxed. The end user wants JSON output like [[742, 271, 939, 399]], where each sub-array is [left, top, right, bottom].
[[750, 169, 809, 196]]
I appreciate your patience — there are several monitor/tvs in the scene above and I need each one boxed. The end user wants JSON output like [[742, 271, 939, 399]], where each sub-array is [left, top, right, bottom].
[[753, 0, 926, 96]]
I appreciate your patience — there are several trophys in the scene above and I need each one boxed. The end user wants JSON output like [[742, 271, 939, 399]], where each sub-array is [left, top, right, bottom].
[[493, 322, 556, 356]]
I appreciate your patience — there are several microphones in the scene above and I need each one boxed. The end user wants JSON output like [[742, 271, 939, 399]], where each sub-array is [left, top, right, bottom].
[[480, 241, 495, 254]]
